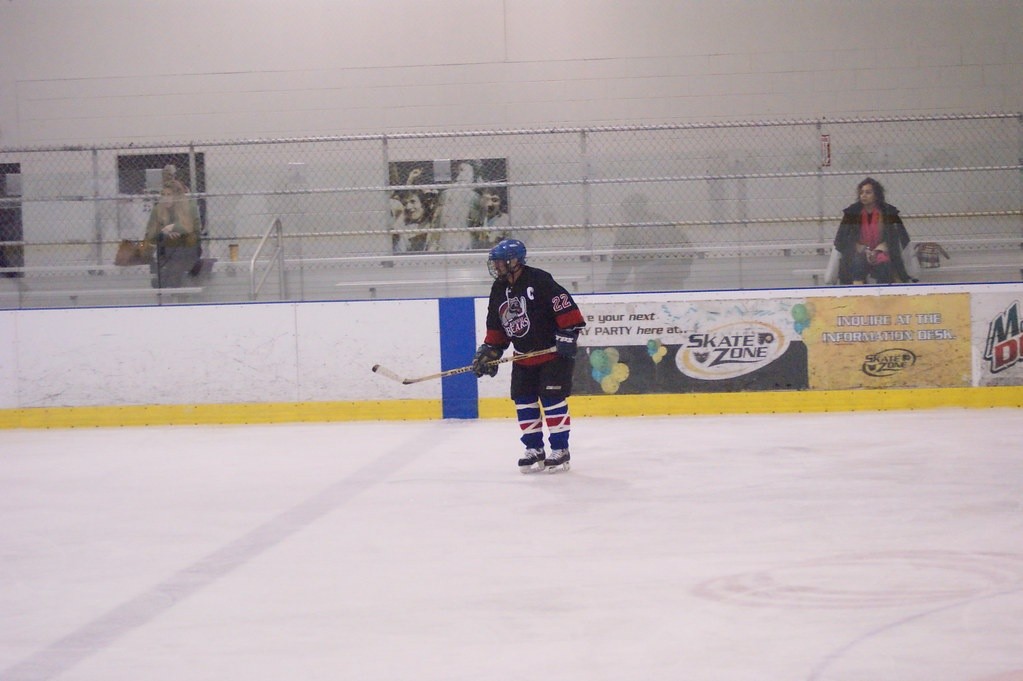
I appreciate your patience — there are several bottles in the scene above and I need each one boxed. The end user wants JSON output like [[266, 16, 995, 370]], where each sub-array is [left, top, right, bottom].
[[865, 246, 874, 264]]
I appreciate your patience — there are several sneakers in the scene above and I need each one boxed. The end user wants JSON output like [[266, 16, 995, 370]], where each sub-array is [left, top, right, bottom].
[[544, 447, 571, 475], [518, 447, 546, 474]]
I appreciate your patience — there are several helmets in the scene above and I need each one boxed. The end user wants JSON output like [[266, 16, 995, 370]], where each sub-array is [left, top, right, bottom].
[[487, 239, 527, 283]]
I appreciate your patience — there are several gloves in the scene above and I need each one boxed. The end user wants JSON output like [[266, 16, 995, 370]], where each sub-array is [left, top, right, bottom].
[[556, 322, 586, 359], [473, 342, 503, 377]]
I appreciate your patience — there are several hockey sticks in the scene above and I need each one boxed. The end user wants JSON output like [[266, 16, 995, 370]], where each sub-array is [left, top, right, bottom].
[[371, 345, 557, 385]]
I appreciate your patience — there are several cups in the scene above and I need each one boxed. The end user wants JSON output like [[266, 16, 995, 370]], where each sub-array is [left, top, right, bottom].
[[229, 244, 239, 261]]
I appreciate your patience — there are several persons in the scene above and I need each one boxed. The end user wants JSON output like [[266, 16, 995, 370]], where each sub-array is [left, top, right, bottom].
[[162, 164, 190, 192], [473, 240, 586, 474], [834, 177, 911, 284], [145, 181, 200, 289], [389, 162, 509, 252]]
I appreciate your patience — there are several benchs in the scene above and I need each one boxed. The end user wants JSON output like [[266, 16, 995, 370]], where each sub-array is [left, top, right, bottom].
[[2, 235, 1022, 310]]
